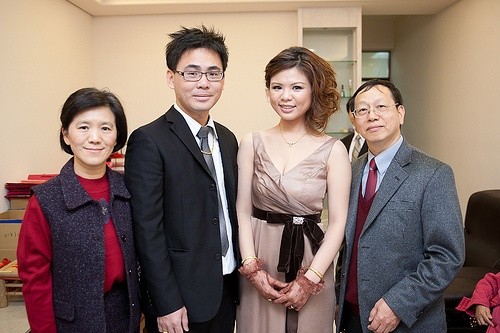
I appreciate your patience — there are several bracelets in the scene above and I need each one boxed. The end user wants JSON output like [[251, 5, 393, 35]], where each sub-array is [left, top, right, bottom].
[[241, 256, 256, 264], [309, 266, 323, 281]]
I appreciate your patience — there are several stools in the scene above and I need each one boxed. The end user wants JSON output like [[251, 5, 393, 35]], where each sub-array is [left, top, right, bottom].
[[0, 260, 23, 308]]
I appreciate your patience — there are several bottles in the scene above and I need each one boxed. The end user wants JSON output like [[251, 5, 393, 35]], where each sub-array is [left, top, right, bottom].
[[340, 84, 345, 98]]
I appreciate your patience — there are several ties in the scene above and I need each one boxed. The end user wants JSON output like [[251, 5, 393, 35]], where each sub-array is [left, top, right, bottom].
[[197, 126, 229, 257], [364, 159, 378, 201], [352, 135, 361, 161]]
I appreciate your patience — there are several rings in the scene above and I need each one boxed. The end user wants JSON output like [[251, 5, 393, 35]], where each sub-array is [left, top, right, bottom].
[[163, 331, 168, 333], [268, 299, 271, 302], [290, 305, 294, 309]]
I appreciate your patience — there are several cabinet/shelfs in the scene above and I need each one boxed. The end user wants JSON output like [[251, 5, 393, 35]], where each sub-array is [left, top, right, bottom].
[[297, 7, 363, 136]]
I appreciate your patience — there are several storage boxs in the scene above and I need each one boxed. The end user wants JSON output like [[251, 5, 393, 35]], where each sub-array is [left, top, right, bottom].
[[0, 210, 25, 261]]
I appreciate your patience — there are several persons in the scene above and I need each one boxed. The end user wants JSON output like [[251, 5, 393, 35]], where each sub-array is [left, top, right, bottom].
[[455, 272, 500, 333], [335, 79, 465, 333], [17, 87, 141, 333], [237, 47, 351, 333], [340, 95, 369, 163], [123, 24, 240, 333]]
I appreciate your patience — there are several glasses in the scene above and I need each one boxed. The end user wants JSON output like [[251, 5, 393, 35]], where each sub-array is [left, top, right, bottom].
[[350, 102, 398, 118], [174, 70, 224, 82]]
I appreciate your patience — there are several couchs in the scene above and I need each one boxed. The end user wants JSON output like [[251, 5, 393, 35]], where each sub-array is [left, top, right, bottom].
[[444, 190, 500, 333]]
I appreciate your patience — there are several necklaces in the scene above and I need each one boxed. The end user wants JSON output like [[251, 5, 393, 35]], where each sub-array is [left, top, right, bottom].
[[279, 123, 310, 148], [200, 133, 215, 154]]
